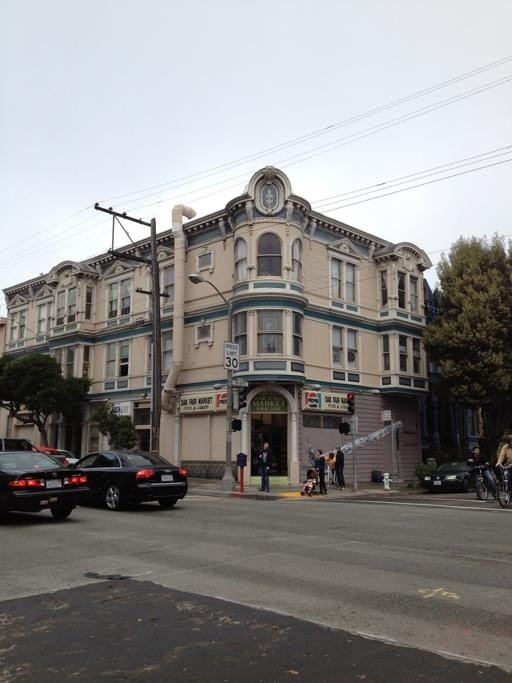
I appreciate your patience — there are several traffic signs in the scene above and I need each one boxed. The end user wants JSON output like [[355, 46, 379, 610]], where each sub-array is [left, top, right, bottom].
[[222, 340, 240, 371]]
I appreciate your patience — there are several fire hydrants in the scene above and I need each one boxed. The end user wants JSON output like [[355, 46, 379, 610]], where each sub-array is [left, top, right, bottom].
[[382, 472, 392, 490]]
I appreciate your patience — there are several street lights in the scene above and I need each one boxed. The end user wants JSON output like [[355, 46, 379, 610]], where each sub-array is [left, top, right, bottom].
[[163, 385, 184, 469], [187, 271, 241, 494]]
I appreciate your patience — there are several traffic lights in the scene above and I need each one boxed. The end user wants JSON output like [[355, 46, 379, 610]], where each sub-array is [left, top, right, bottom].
[[237, 388, 248, 409], [345, 391, 356, 413]]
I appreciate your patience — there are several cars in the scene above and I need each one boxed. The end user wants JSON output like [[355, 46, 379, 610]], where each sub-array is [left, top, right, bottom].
[[421, 455, 471, 491], [70, 448, 190, 512], [34, 446, 79, 464], [0, 450, 92, 521]]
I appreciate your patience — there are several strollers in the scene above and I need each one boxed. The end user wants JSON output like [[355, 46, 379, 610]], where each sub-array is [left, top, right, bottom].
[[299, 467, 320, 496]]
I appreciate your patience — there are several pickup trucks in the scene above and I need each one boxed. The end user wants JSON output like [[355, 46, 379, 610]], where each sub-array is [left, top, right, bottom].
[[0, 437, 69, 468]]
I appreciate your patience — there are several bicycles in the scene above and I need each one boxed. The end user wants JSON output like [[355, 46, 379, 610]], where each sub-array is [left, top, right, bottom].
[[325, 464, 339, 491], [495, 462, 512, 508], [471, 464, 497, 500]]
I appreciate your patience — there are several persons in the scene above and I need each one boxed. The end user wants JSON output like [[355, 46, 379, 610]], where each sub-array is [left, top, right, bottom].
[[257, 441, 271, 491], [302, 447, 344, 496], [464, 431, 511, 500]]
